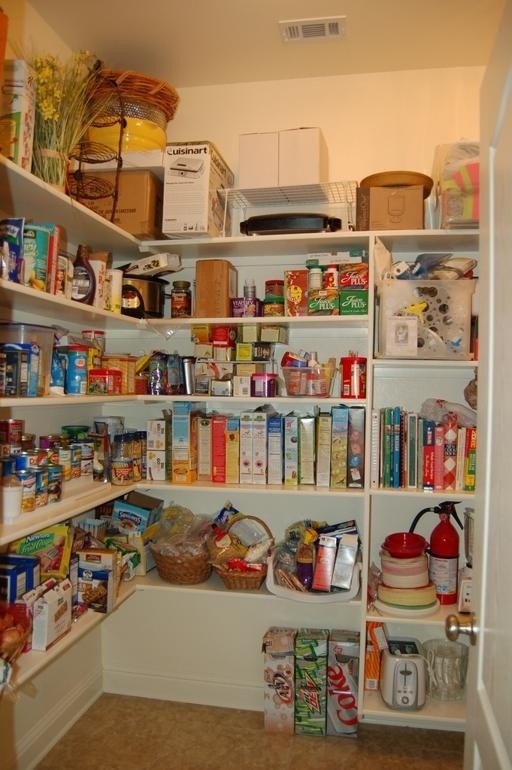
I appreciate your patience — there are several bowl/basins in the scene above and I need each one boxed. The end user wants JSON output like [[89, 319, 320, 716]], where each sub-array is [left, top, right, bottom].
[[381, 532, 430, 559]]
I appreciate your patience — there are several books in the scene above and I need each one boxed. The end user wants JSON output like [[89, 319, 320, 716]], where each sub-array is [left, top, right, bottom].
[[371, 405, 477, 492]]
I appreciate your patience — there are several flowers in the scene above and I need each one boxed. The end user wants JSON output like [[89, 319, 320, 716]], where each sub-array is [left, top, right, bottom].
[[23, 47, 119, 189]]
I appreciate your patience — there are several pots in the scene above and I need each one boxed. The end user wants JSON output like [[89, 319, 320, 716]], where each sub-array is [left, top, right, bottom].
[[120, 274, 170, 319]]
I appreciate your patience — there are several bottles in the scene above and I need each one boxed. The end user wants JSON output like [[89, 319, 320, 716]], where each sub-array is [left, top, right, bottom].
[[0, 475, 23, 525], [182, 358, 194, 395], [62, 243, 97, 305], [243, 279, 256, 297]]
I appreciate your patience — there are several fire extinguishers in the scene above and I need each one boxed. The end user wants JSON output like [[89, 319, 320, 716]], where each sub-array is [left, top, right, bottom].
[[431, 500, 461, 604]]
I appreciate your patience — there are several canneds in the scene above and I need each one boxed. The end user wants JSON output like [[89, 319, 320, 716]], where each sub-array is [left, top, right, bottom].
[[111, 431, 146, 485], [327, 662, 358, 734], [0, 419, 82, 513], [265, 280, 284, 299], [262, 300, 284, 316], [170, 281, 190, 318]]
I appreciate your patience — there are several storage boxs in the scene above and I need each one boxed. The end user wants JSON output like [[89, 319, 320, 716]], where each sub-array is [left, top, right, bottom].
[[372, 278, 475, 363], [161, 137, 234, 238], [192, 257, 241, 323], [236, 125, 330, 202], [66, 170, 162, 240], [355, 185, 426, 232], [0, 321, 57, 397]]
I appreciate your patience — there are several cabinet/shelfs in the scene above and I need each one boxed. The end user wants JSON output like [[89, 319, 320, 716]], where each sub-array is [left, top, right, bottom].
[[0, 148, 143, 702], [360, 223, 494, 735], [141, 233, 371, 610]]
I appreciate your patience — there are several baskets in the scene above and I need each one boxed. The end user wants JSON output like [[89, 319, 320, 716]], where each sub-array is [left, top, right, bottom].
[[209, 515, 276, 591], [148, 544, 213, 586]]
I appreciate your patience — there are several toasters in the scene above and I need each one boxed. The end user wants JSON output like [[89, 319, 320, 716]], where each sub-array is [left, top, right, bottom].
[[379, 636, 428, 713]]
